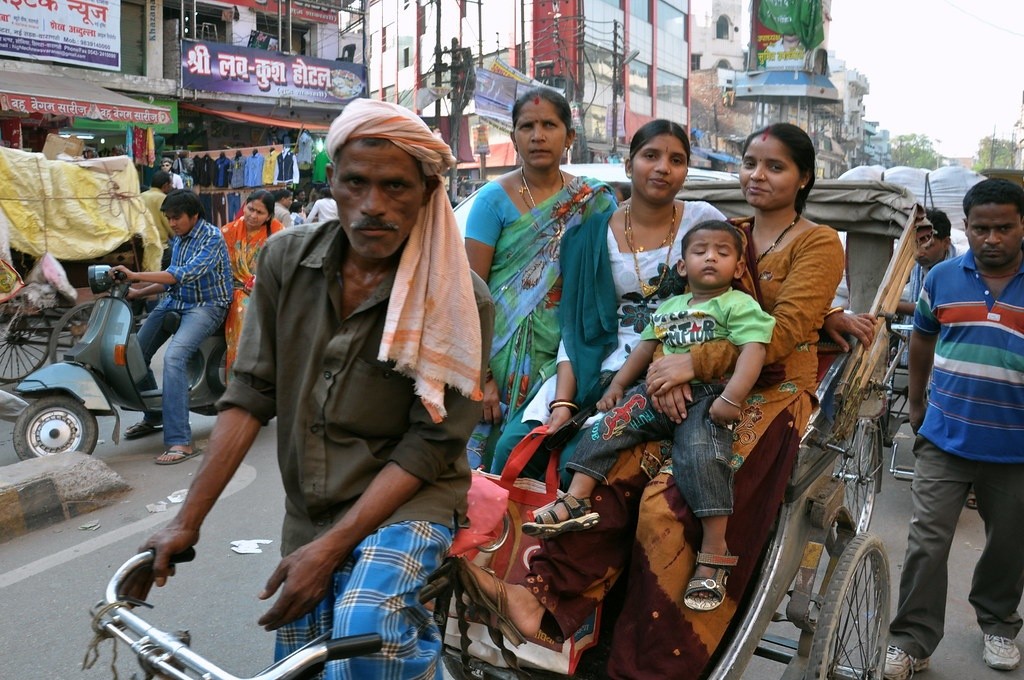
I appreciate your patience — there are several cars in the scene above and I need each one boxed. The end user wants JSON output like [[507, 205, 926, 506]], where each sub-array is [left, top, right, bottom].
[[451, 160, 723, 245]]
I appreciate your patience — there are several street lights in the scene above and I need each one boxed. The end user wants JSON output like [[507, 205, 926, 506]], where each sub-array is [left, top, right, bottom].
[[612, 49, 642, 154]]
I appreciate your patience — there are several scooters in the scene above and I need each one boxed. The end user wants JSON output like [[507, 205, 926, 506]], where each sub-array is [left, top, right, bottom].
[[11, 262, 230, 461]]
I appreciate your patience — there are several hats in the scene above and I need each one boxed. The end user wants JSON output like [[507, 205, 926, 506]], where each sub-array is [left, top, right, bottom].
[[162, 157, 173, 163]]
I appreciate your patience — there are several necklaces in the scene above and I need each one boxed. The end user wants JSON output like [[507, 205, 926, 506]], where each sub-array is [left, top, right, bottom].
[[518, 163, 566, 209], [751, 212, 800, 265], [625, 198, 677, 296], [981, 268, 1021, 278]]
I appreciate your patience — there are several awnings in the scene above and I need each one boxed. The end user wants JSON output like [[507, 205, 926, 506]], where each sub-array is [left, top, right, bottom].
[[182, 103, 331, 130], [1, 69, 171, 126], [588, 142, 741, 169]]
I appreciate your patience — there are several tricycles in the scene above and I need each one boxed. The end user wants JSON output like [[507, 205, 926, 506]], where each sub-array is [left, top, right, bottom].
[[0, 145, 163, 386], [830, 167, 990, 535], [76, 177, 939, 680]]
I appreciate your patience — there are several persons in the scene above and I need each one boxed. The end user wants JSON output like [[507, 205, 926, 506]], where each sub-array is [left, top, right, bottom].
[[895, 209, 976, 508], [876, 177, 1024, 680], [465, 86, 618, 468], [454, 124, 846, 680], [144, 157, 194, 191], [137, 97, 495, 680], [123, 171, 338, 464], [520, 219, 775, 613], [489, 120, 877, 480]]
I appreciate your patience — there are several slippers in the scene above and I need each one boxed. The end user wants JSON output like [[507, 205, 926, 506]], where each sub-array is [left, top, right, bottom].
[[156, 450, 200, 465], [460, 555, 527, 648], [123, 422, 165, 437]]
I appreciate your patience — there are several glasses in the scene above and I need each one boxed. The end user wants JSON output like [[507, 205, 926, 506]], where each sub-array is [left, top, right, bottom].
[[162, 164, 169, 167]]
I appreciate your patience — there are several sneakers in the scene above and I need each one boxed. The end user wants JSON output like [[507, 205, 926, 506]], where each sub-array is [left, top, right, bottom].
[[983, 633, 1021, 670], [884, 645, 929, 680]]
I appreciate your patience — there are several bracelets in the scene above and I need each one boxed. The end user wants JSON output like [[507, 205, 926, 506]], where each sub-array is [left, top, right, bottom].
[[720, 392, 741, 406], [824, 306, 843, 318]]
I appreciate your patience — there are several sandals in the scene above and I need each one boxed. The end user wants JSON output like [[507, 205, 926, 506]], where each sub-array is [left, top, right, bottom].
[[521, 492, 599, 539], [684, 549, 739, 610], [967, 491, 977, 509]]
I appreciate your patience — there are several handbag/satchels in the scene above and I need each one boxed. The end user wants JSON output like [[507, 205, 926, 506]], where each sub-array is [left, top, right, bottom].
[[449, 424, 560, 583]]
